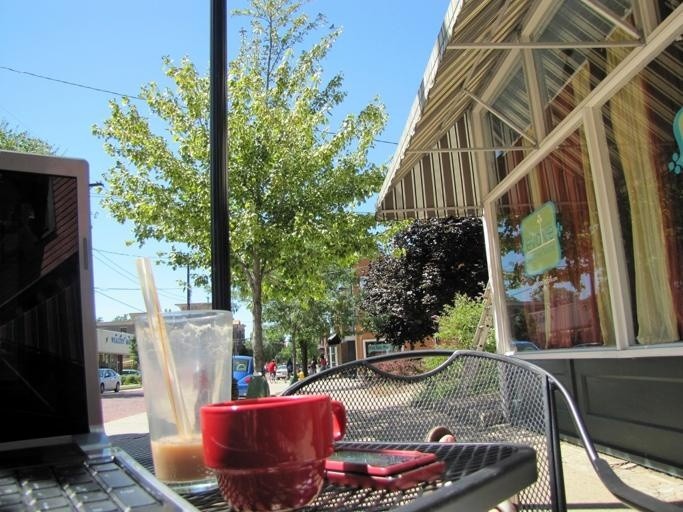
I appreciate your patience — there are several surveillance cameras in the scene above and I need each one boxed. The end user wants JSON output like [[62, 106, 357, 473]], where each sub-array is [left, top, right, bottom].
[[495, 151, 502, 158]]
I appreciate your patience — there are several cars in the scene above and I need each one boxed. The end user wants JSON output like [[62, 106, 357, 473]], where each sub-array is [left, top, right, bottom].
[[276, 365, 287, 380], [231, 354, 265, 398], [96, 368, 121, 393], [512, 338, 542, 352], [121, 369, 138, 375]]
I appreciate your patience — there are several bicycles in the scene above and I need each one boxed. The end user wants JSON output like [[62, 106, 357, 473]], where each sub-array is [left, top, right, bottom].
[[269, 372, 277, 384]]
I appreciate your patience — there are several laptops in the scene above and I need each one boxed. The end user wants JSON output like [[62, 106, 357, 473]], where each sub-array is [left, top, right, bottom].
[[0, 151, 202, 512]]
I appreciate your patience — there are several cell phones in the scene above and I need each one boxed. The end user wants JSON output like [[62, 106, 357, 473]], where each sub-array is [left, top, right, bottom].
[[323, 447, 448, 491]]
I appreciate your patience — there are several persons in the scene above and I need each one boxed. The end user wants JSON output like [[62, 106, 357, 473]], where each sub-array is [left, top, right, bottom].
[[319, 355, 326, 371], [267, 359, 277, 380], [285, 357, 292, 380], [309, 358, 316, 373]]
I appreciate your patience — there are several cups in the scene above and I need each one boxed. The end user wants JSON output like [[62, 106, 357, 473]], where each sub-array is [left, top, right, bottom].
[[197, 392, 348, 512], [130, 308, 237, 496]]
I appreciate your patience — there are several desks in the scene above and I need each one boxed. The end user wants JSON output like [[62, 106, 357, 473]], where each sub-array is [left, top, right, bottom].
[[279, 348, 683, 512], [105, 432, 536, 511]]
